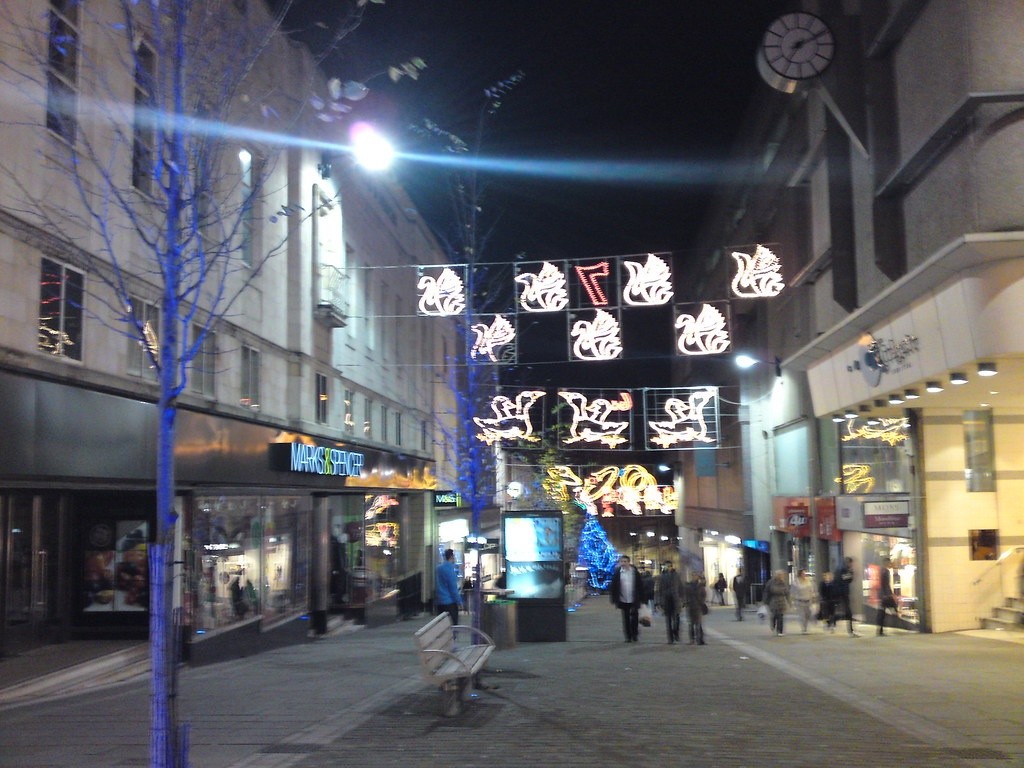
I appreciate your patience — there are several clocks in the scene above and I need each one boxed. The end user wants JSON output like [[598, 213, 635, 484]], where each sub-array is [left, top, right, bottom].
[[756, 13, 836, 94]]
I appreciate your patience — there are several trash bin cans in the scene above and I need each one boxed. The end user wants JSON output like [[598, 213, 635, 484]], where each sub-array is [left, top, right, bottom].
[[481, 589, 518, 649]]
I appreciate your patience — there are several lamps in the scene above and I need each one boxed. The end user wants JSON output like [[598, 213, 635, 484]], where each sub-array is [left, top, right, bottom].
[[926, 382, 944, 393], [873, 399, 887, 407], [949, 373, 968, 385], [844, 409, 859, 418], [977, 363, 997, 376], [905, 390, 920, 399], [832, 415, 846, 423], [889, 394, 903, 404], [860, 405, 872, 412], [867, 417, 881, 425]]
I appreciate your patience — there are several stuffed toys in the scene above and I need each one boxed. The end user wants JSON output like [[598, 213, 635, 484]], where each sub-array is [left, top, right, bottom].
[[85, 519, 149, 608]]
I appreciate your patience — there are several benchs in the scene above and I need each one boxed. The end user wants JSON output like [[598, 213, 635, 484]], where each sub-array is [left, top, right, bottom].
[[415, 611, 496, 716]]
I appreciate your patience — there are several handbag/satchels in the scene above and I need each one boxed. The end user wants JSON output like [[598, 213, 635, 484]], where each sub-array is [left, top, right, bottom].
[[757, 605, 768, 624], [701, 604, 708, 615], [638, 603, 653, 627]]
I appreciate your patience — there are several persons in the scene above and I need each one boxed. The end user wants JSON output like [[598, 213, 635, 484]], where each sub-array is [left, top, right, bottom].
[[876, 557, 911, 637], [762, 570, 792, 637], [685, 574, 708, 645], [789, 570, 814, 635], [827, 556, 862, 638], [610, 555, 642, 644], [716, 572, 727, 605], [227, 570, 259, 624], [733, 567, 747, 621], [540, 527, 559, 548], [709, 578, 723, 606], [435, 549, 461, 641], [639, 571, 655, 627], [654, 560, 687, 645]]
